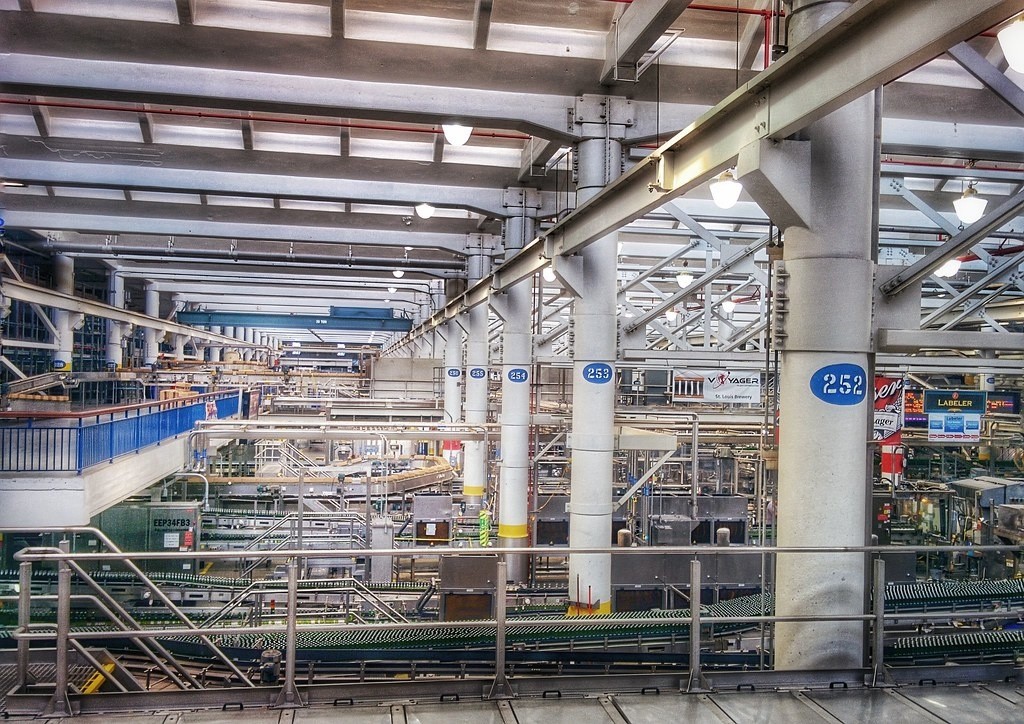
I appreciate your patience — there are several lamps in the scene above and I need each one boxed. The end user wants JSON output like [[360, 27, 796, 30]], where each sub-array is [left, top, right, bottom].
[[710, 168, 743, 210], [385, 203, 434, 302], [935, 260, 961, 297], [543, 244, 735, 321], [442, 125, 473, 148], [996, 13, 1024, 74], [953, 180, 988, 224]]
